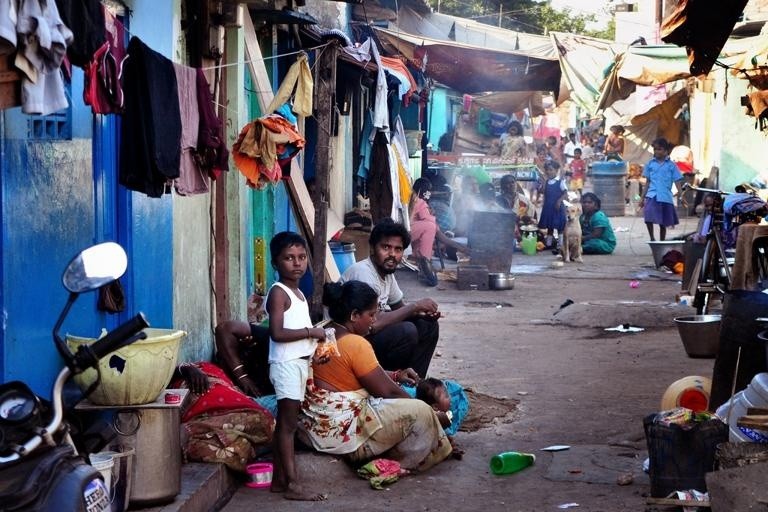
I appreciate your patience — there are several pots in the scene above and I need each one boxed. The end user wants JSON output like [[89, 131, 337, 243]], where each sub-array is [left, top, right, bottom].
[[489, 271, 516, 290]]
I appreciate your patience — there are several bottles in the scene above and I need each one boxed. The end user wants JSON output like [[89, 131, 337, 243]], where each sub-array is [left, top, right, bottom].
[[521, 231, 526, 240], [489, 451, 537, 474], [527, 232, 533, 239], [534, 232, 536, 237]]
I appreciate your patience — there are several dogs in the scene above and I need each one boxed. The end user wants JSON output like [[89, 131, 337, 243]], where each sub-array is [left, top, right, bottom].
[[560, 205, 583, 263]]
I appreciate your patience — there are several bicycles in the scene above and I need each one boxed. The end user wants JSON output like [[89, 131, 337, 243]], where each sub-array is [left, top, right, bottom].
[[680, 182, 754, 315]]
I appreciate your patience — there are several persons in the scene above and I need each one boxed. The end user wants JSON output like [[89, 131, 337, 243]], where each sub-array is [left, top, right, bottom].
[[579, 193, 616, 254], [263, 231, 327, 502], [538, 161, 568, 249], [497, 120, 526, 160], [452, 175, 480, 238], [409, 177, 461, 262], [170, 361, 209, 396], [339, 218, 442, 379], [603, 125, 626, 161], [496, 174, 537, 238], [210, 316, 272, 396], [703, 194, 721, 211], [414, 378, 452, 413], [548, 132, 604, 163], [293, 280, 452, 472], [477, 182, 502, 211], [569, 148, 585, 197], [639, 137, 684, 242], [529, 148, 546, 203]]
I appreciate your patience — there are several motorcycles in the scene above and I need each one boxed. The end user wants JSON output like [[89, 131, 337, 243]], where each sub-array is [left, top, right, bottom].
[[0, 241, 150, 512]]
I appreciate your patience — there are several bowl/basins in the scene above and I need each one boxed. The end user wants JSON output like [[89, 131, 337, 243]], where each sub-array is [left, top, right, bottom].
[[674, 315, 723, 359], [646, 241, 686, 270]]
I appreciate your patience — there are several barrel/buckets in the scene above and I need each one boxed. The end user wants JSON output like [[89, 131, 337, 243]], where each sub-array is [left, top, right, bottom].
[[327, 241, 356, 275], [722, 373, 768, 447], [90, 454, 115, 503], [108, 409, 180, 506], [660, 376, 713, 414], [593, 175, 625, 216]]
